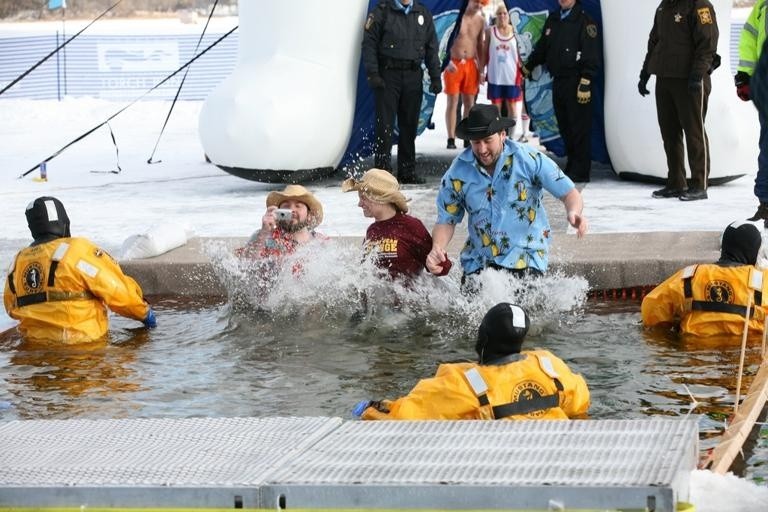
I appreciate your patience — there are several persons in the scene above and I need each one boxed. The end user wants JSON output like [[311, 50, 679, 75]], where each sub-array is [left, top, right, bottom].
[[641, 219, 768, 345], [355, 302, 591, 420], [522, 1, 598, 181], [443, 1, 537, 149], [234, 183, 333, 326], [425, 103, 585, 315], [735, 0, 768, 220], [638, 0, 722, 201], [363, 0, 442, 184], [3, 194, 158, 346], [340, 168, 451, 325]]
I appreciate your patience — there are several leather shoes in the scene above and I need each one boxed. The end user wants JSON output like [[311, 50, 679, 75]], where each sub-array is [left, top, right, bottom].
[[397, 174, 426, 184]]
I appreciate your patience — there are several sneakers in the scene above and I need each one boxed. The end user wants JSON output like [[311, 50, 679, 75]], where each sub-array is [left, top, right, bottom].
[[680, 188, 707, 200], [447, 138, 456, 148], [653, 186, 683, 198], [464, 139, 471, 147]]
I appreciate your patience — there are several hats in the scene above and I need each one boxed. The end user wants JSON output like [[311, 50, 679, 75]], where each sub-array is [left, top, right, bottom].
[[456, 104, 516, 140], [26, 197, 70, 245], [719, 220, 762, 265], [475, 303, 529, 364], [266, 184, 323, 229], [342, 168, 408, 214]]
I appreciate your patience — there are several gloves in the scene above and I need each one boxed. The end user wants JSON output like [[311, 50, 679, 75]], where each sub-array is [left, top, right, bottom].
[[429, 79, 441, 94], [368, 76, 386, 89], [735, 71, 753, 101], [638, 79, 649, 97], [577, 77, 591, 104]]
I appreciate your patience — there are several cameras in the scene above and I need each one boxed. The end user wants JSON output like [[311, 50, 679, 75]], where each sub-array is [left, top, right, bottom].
[[275, 209, 291, 220]]
